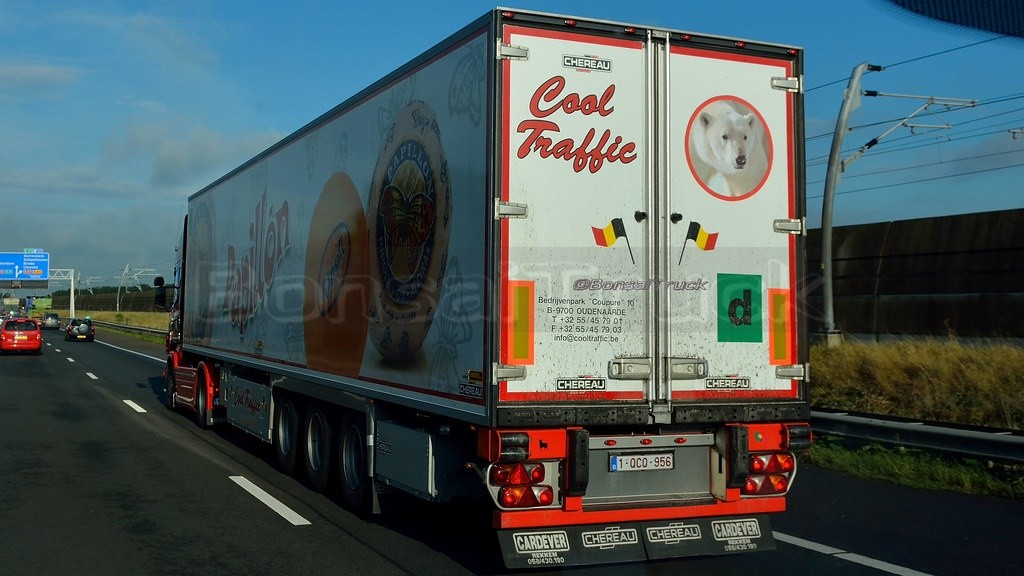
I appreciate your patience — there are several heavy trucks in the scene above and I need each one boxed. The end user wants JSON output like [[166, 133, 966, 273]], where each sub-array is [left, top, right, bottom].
[[151, 5, 814, 576]]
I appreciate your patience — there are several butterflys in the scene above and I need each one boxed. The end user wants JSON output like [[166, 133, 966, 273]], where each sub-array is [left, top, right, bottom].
[[380, 167, 434, 274]]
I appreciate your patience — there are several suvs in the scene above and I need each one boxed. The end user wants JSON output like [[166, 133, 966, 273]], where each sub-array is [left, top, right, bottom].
[[65, 318, 95, 343]]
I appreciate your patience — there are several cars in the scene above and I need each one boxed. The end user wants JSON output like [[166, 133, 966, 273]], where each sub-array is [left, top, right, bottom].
[[0, 318, 42, 355], [40, 313, 61, 330]]
